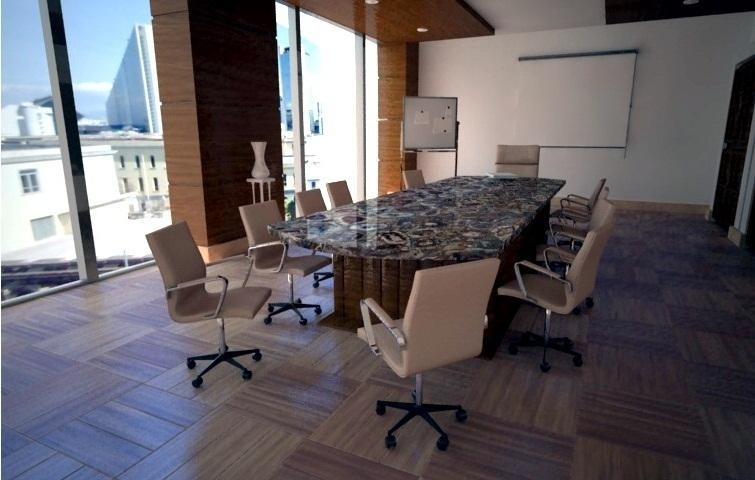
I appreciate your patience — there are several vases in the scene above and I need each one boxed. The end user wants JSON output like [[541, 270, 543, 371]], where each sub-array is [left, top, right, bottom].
[[250, 140, 270, 178]]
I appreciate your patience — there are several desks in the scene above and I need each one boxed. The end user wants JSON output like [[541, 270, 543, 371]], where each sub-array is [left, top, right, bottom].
[[267, 176, 567, 325]]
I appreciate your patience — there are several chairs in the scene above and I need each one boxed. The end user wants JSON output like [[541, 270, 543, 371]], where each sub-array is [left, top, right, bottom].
[[496, 175, 618, 371], [147, 223, 271, 392], [295, 189, 335, 289], [240, 200, 333, 326], [354, 253, 504, 453], [495, 146, 540, 184], [326, 179, 353, 208], [400, 168, 425, 190]]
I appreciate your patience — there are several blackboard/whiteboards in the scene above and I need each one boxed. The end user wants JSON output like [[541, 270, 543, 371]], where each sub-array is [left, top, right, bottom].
[[403, 96, 457, 150]]
